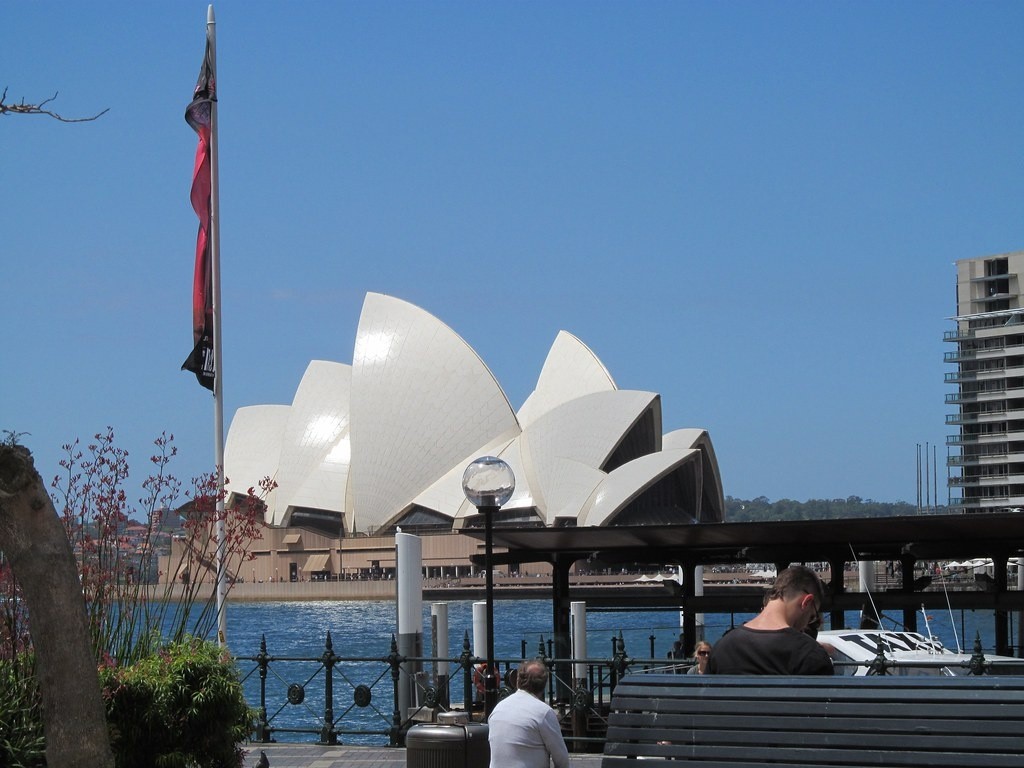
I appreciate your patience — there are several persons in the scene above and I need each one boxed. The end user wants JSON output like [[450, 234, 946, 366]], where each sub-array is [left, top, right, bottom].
[[702, 566, 836, 677], [687, 641, 711, 675], [667, 633, 686, 674], [487, 658, 570, 768]]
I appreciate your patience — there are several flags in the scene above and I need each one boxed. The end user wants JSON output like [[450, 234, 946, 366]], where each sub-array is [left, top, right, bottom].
[[179, 26, 217, 393]]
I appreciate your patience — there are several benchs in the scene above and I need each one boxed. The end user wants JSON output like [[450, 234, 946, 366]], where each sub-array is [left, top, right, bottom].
[[601, 675, 1024, 768]]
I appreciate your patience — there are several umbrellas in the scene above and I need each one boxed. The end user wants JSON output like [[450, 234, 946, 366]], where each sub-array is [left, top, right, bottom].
[[633, 573, 679, 582], [943, 560, 1017, 567], [749, 569, 777, 578]]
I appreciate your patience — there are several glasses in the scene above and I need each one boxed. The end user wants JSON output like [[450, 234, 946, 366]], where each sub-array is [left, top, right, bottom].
[[803, 589, 821, 625], [698, 651, 710, 656]]
